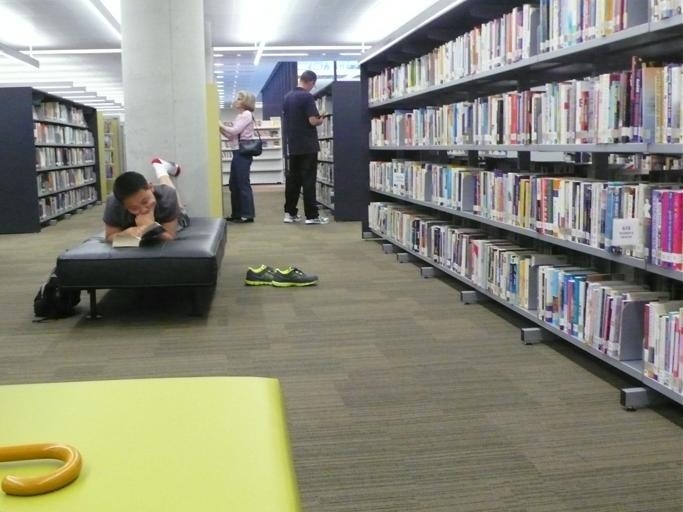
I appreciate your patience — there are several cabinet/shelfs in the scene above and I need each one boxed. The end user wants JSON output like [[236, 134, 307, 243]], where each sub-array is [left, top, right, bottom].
[[220, 116, 284, 187], [0, 87, 121, 233]]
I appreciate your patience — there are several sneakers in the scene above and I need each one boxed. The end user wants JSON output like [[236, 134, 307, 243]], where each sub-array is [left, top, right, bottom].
[[273, 266, 318, 287], [244, 265, 274, 286], [285, 213, 301, 222], [305, 215, 329, 225], [226, 216, 234, 221], [235, 217, 254, 223]]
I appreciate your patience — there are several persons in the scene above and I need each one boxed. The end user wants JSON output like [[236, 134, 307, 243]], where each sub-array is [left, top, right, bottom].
[[218, 90, 256, 224], [281, 70, 329, 225], [101, 157, 189, 244]]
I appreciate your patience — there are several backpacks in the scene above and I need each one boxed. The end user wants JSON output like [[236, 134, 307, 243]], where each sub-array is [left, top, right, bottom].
[[34, 269, 80, 318]]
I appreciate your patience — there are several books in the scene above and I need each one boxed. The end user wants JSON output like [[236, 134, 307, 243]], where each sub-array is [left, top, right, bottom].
[[311, 95, 335, 211], [220, 119, 280, 159], [103, 119, 115, 179], [111, 221, 168, 249], [366, 52, 446, 258], [31, 98, 96, 222], [535, 0, 683, 396], [431, 2, 564, 309]]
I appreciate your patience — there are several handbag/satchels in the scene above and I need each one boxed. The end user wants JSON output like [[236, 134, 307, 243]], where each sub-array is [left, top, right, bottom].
[[238, 139, 263, 155]]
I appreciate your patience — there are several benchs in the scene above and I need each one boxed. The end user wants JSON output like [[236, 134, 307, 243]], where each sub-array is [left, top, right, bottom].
[[55, 216, 227, 321]]
